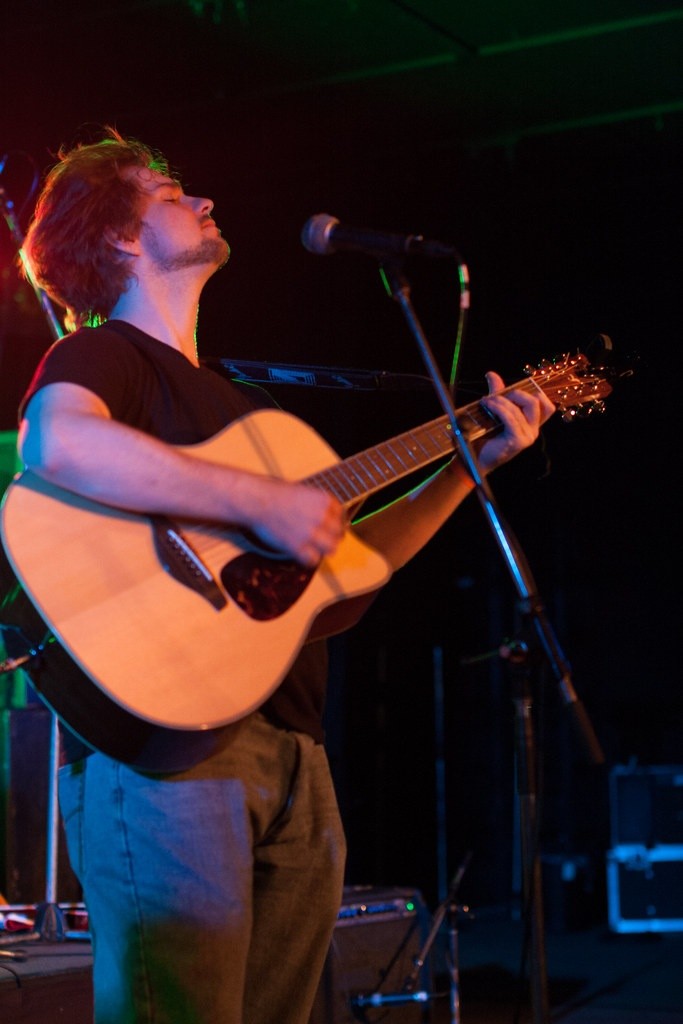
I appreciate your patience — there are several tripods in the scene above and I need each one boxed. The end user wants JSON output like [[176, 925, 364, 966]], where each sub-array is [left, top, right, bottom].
[[0, 715, 94, 949]]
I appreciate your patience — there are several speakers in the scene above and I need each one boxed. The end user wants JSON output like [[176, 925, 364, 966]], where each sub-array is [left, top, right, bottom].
[[308, 884, 437, 1024]]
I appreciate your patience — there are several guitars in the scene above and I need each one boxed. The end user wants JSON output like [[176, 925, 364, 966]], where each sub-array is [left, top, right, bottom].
[[0, 325, 643, 780]]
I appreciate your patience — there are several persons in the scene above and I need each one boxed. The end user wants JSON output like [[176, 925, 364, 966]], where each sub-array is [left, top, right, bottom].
[[14, 132, 558, 1024]]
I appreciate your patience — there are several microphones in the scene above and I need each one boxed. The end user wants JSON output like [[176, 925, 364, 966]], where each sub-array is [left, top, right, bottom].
[[303, 214, 458, 260], [350, 990, 436, 1010]]
